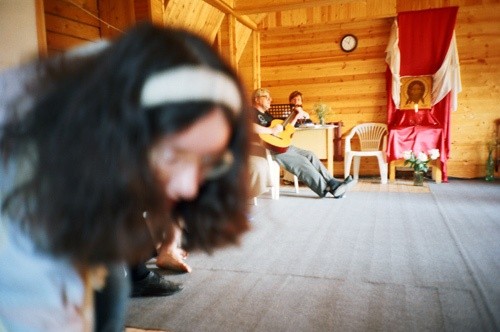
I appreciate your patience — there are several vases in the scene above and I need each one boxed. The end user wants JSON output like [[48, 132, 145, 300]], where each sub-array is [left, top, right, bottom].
[[318, 114, 325, 124], [484, 154, 496, 182], [410, 160, 429, 187]]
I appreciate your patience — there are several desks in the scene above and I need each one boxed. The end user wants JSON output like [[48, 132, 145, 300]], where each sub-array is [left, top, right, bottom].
[[283, 125, 340, 191]]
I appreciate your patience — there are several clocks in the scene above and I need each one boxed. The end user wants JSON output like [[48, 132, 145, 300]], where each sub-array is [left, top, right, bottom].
[[341, 35, 358, 52]]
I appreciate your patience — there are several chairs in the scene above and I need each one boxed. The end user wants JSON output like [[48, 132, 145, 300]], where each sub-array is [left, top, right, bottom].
[[344, 123, 389, 184], [249, 143, 299, 206]]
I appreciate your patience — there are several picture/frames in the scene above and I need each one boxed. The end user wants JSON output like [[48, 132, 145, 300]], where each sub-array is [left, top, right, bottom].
[[399, 74, 435, 110]]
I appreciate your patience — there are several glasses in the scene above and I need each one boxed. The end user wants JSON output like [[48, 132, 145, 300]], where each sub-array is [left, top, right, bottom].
[[153, 139, 235, 180], [258, 95, 270, 100]]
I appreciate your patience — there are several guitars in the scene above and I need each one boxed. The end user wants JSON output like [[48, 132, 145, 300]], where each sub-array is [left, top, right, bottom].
[[255, 104, 303, 154]]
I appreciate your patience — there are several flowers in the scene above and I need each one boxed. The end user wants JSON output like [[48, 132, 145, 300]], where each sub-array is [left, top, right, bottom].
[[402, 149, 442, 170], [477, 122, 499, 157], [313, 103, 330, 117]]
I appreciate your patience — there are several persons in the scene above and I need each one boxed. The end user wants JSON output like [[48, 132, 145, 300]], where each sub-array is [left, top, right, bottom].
[[282, 91, 310, 119], [0, 21, 250, 331], [408, 83, 423, 101], [250, 88, 355, 199]]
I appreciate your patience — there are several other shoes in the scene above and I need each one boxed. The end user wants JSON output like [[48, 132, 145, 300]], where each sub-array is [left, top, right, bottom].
[[328, 175, 352, 198], [132, 271, 183, 298]]
